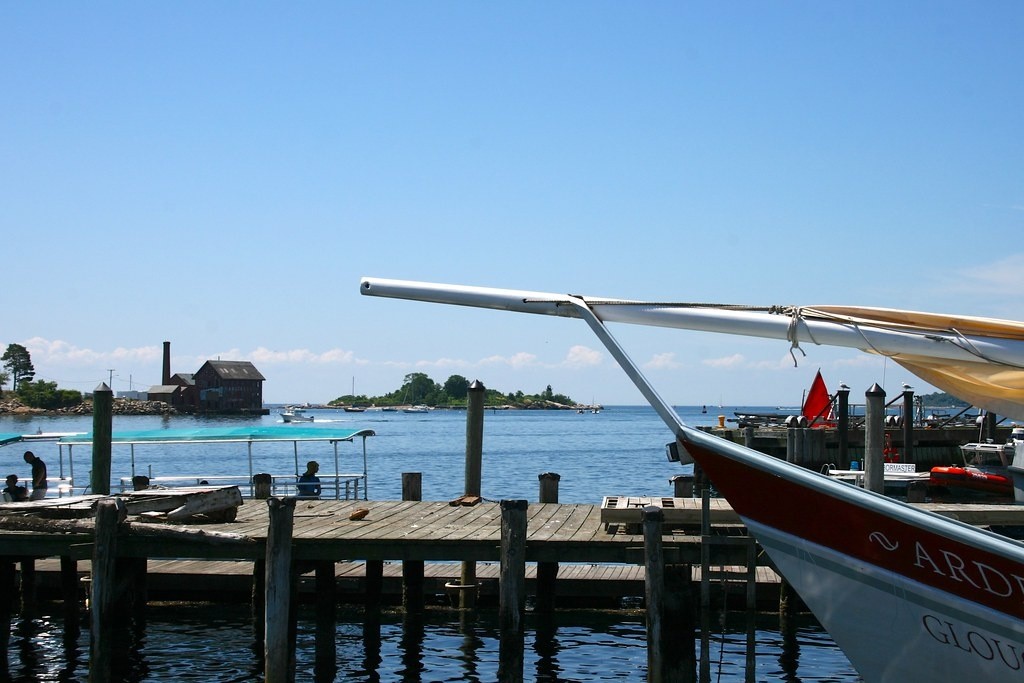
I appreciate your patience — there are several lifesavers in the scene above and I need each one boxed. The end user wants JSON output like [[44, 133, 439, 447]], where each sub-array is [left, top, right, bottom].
[[884, 448, 899, 462]]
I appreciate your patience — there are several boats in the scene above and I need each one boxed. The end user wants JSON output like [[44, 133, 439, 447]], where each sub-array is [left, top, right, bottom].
[[279, 408, 315, 423], [358, 278, 1024, 683], [382, 406, 398, 412], [343, 406, 367, 412], [401, 404, 436, 413], [576, 408, 600, 414]]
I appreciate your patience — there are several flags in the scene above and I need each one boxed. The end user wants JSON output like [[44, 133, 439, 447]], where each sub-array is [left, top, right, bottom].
[[804, 371, 836, 428]]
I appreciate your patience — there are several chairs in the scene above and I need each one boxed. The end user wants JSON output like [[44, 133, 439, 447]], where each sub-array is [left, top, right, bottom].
[[1, 490, 14, 503]]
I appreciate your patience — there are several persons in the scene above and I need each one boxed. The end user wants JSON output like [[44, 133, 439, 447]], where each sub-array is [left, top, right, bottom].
[[4, 474, 29, 502], [24, 451, 47, 500], [298, 460, 322, 500]]
